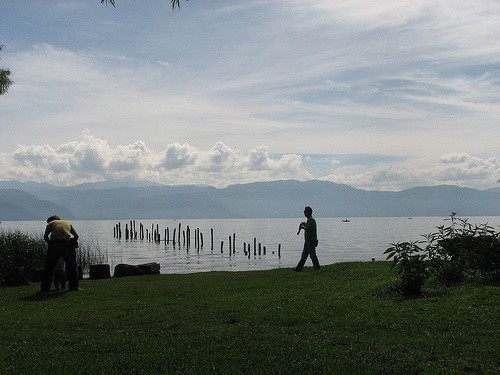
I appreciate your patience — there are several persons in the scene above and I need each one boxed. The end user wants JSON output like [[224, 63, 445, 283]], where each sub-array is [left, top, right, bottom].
[[291, 206, 322, 272], [345, 219, 347, 221], [52, 256, 67, 290], [35, 215, 84, 296]]
